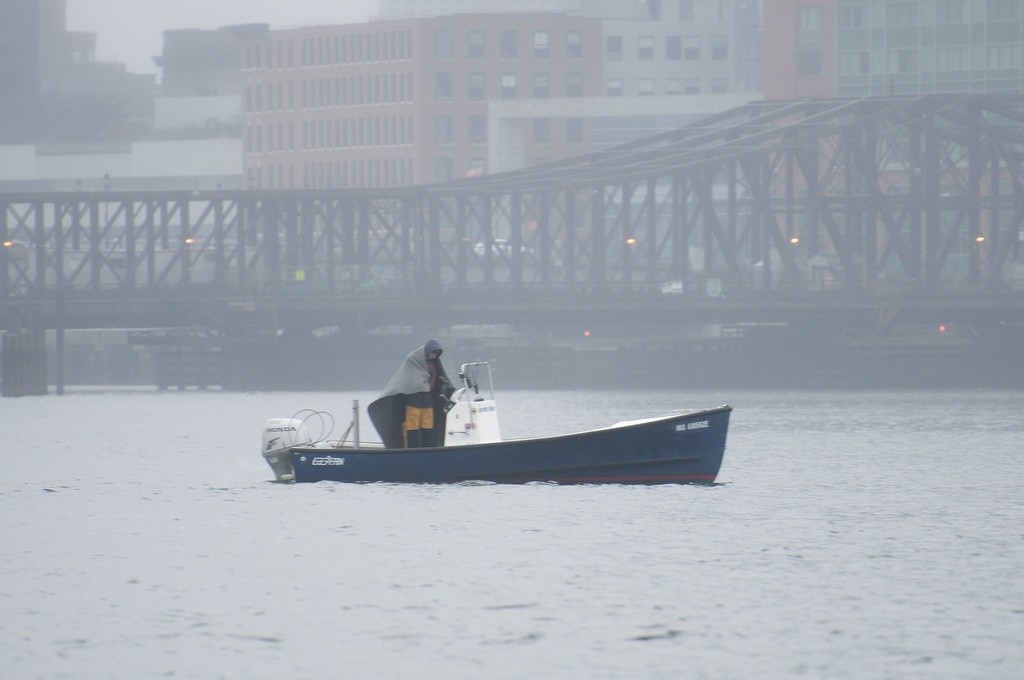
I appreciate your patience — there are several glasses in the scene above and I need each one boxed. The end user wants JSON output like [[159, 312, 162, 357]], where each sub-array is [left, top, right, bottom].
[[432, 351, 440, 355]]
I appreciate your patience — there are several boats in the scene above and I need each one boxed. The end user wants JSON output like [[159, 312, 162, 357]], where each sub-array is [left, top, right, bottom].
[[261, 360, 733, 485]]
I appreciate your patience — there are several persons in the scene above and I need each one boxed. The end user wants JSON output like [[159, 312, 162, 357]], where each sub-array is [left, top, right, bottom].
[[400, 338, 444, 449]]
